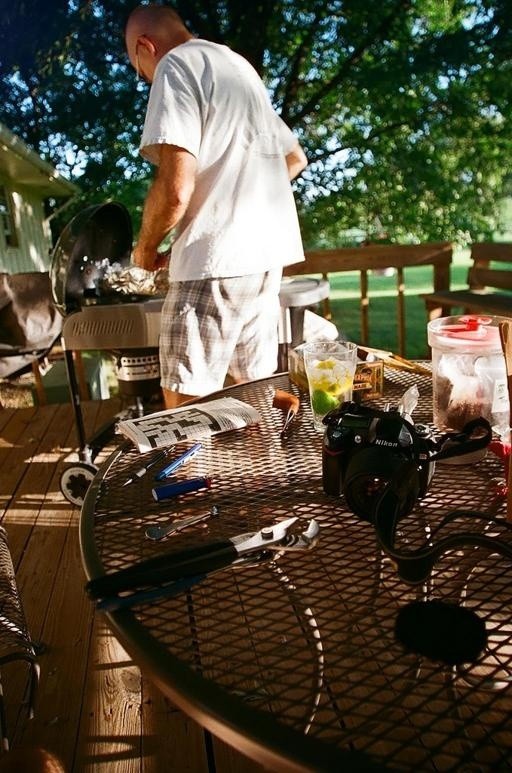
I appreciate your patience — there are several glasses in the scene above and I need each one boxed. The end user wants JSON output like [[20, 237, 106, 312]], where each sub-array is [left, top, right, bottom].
[[133, 33, 150, 86]]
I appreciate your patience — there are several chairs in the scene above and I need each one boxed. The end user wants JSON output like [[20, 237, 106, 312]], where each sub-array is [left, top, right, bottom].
[[1, 271, 87, 407]]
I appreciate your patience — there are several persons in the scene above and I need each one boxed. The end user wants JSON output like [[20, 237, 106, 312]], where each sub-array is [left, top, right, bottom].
[[125, 3, 309, 504]]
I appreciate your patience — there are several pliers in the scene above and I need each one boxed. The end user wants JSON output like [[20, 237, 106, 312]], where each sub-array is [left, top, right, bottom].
[[84, 517, 320, 612]]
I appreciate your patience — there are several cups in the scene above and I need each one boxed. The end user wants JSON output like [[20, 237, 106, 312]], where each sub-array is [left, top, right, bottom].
[[306, 344, 358, 428]]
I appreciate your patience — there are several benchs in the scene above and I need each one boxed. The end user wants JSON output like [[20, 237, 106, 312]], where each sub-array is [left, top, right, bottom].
[[418, 240, 512, 358]]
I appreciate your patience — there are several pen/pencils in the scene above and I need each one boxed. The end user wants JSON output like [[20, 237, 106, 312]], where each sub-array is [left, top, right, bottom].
[[120, 443, 203, 488]]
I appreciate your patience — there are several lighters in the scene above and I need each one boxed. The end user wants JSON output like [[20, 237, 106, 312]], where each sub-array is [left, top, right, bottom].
[[152, 472, 212, 500]]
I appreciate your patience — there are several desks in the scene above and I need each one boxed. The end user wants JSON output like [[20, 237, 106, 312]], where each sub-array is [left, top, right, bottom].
[[61, 299, 165, 511], [77, 362, 512, 772]]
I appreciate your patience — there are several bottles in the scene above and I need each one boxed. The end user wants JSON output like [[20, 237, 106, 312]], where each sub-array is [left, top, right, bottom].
[[430, 310, 511, 449]]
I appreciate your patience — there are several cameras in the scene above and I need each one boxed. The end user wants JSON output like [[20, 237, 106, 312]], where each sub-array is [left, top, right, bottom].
[[322, 400, 438, 525]]
